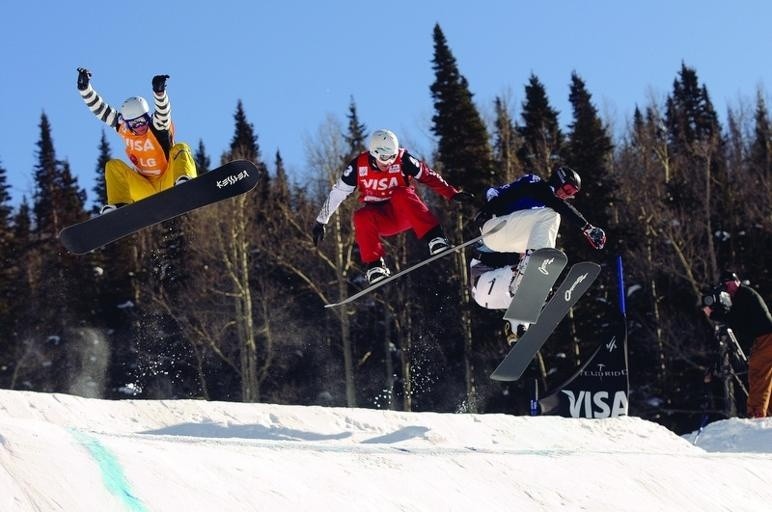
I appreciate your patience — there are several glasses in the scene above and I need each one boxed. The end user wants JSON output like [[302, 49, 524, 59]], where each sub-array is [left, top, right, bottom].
[[128, 117, 149, 128], [376, 154, 398, 165]]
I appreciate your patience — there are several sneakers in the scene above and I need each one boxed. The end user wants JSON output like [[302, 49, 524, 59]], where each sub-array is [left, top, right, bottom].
[[368, 261, 387, 279], [503, 322, 524, 342], [428, 233, 448, 250]]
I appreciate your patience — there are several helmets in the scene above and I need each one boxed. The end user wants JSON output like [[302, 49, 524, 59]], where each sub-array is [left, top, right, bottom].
[[549, 167, 581, 196], [121, 97, 150, 121], [369, 128, 399, 159]]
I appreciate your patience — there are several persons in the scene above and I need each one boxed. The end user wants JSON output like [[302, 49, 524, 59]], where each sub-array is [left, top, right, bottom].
[[56, 67, 259, 256], [308, 129, 505, 325], [703, 270, 772, 418], [466, 247, 602, 382], [463, 165, 607, 326]]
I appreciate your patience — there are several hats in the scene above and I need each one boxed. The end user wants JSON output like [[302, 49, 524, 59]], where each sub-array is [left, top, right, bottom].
[[720, 271, 739, 283]]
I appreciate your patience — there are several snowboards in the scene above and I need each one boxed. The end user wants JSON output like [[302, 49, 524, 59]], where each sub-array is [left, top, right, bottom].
[[502, 248, 568, 324], [60, 157, 259, 257], [490, 261, 601, 382], [324, 221, 508, 308]]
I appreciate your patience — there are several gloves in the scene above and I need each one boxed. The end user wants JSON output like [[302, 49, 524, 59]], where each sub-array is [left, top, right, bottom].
[[312, 222, 324, 246], [455, 190, 474, 203], [583, 223, 606, 249], [152, 75, 170, 92], [76, 67, 93, 90]]
